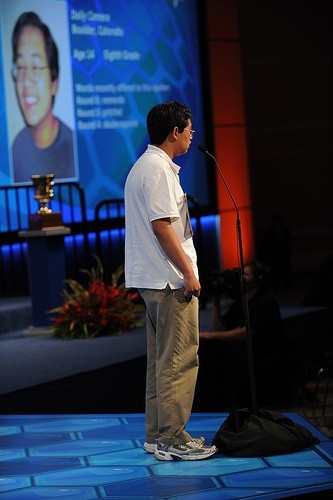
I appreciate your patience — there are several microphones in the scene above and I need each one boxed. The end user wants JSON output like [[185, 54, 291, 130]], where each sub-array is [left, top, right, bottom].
[[198, 143, 240, 222]]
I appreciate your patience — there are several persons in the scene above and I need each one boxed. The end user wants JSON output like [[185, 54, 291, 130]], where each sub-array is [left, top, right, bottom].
[[120, 102, 222, 463], [7, 11, 74, 182], [197, 261, 304, 410]]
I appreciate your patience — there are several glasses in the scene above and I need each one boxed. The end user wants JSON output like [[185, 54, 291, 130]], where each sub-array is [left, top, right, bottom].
[[183, 127, 195, 139], [10, 60, 51, 83]]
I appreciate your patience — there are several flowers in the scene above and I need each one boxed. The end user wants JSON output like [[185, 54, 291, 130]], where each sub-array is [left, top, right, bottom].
[[44, 252, 148, 341]]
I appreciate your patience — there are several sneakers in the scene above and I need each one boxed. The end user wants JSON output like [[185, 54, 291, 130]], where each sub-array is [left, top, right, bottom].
[[143, 437, 205, 453], [153, 440, 218, 461]]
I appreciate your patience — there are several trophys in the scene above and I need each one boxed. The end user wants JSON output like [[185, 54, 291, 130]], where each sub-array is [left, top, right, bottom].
[[31, 172, 56, 215]]
